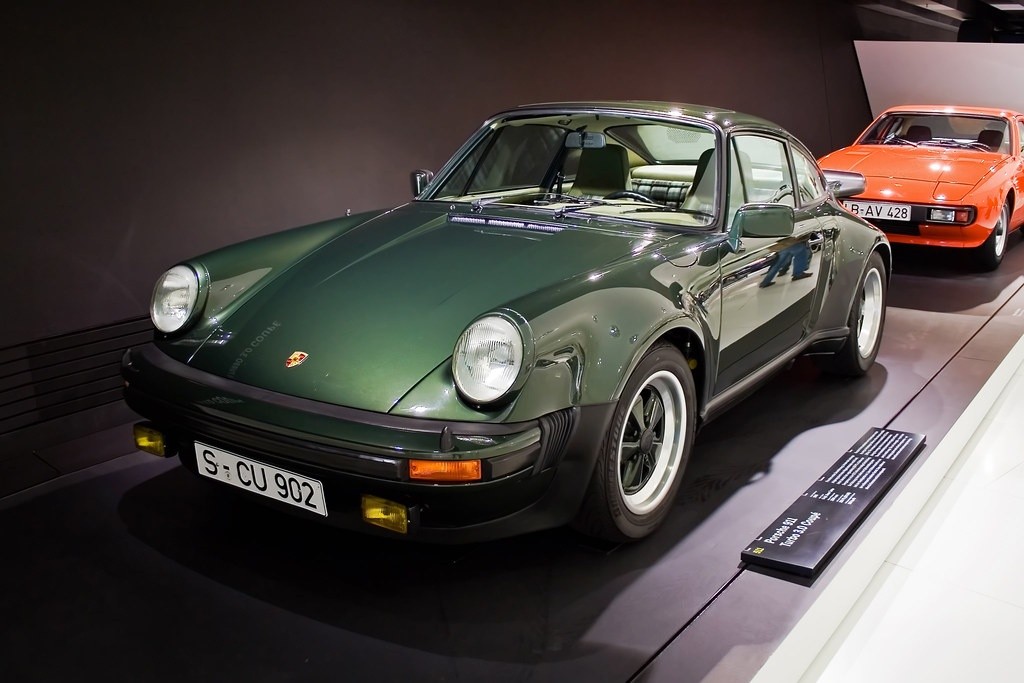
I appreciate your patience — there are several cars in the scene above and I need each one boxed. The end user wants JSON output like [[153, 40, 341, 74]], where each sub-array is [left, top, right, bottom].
[[120, 100, 892, 543], [817, 104, 1024, 270]]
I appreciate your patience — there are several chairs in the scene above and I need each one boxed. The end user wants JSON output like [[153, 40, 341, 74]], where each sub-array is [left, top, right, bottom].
[[682, 148, 754, 226], [978, 130, 1004, 147], [568, 144, 638, 212], [906, 126, 932, 142]]
